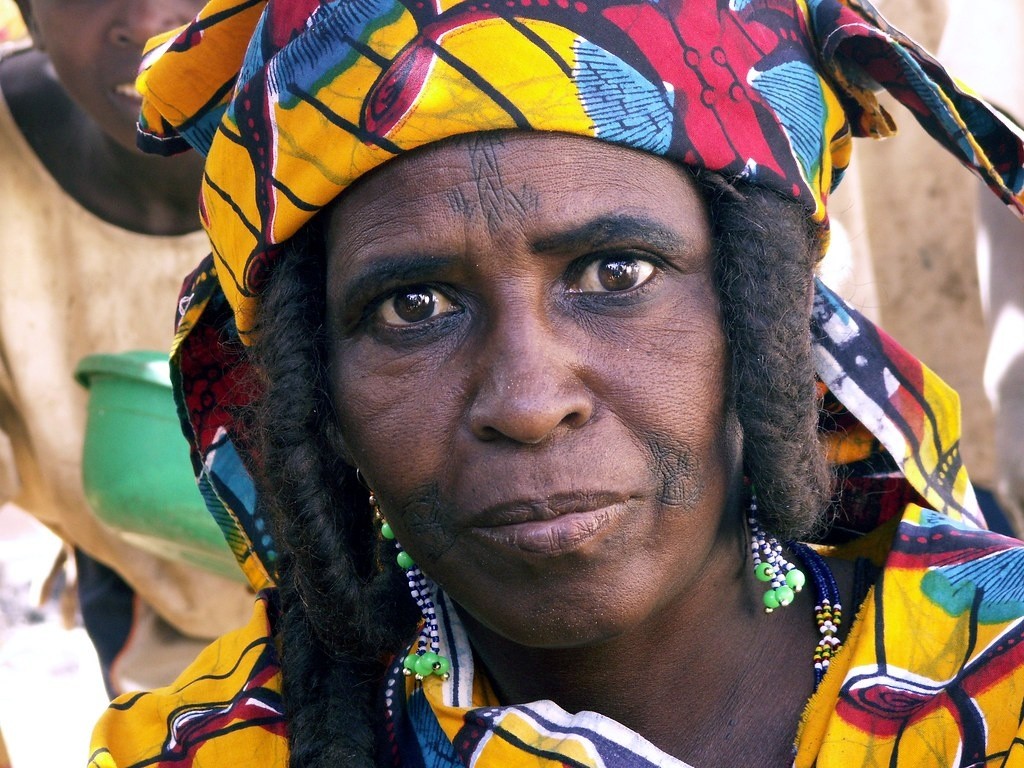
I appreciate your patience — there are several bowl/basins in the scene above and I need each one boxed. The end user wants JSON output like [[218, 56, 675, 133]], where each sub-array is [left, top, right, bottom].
[[71, 349, 252, 583]]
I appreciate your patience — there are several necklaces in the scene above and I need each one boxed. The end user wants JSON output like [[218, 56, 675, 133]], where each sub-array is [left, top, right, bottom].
[[784, 539, 842, 693]]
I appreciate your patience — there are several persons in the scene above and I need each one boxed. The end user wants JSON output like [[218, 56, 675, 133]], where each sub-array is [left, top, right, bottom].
[[78, 0, 1024, 768], [0, 0, 264, 699]]
